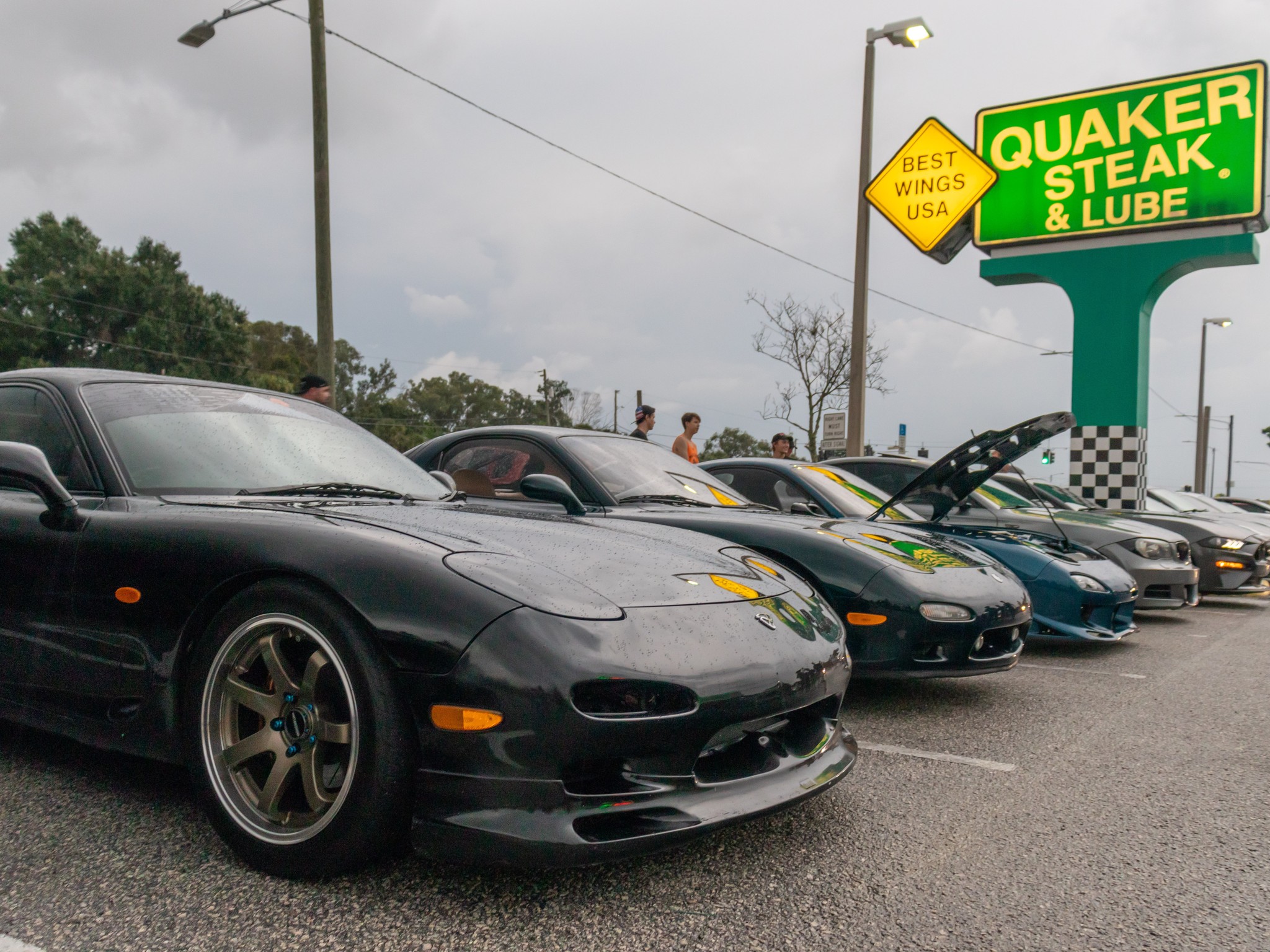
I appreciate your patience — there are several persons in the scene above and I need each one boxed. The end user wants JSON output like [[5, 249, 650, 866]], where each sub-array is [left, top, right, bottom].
[[627, 405, 655, 442], [294, 374, 333, 405], [780, 439, 798, 460], [671, 412, 702, 464], [769, 433, 795, 460]]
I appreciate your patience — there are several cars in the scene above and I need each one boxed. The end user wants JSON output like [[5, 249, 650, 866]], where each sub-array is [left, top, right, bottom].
[[398, 423, 1028, 689], [696, 408, 1143, 656], [3, 368, 855, 885], [812, 455, 1202, 616], [1022, 477, 1270, 596]]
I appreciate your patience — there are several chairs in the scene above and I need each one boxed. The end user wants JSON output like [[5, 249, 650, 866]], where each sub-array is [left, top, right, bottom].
[[451, 468, 497, 500]]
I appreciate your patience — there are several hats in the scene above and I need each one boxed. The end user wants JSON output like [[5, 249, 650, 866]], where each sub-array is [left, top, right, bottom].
[[772, 433, 793, 442], [789, 441, 798, 449], [631, 405, 655, 424], [295, 376, 329, 395]]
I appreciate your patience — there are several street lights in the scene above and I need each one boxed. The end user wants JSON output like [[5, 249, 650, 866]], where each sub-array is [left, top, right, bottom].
[[1193, 312, 1234, 494], [172, 0, 344, 411], [844, 13, 934, 455]]
